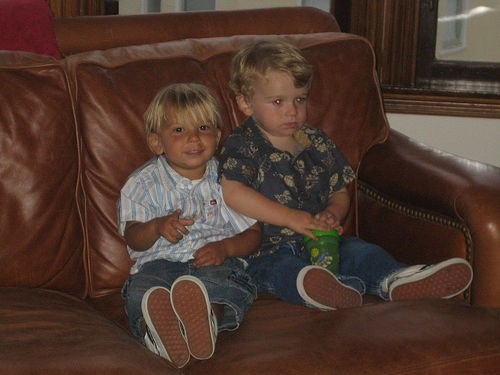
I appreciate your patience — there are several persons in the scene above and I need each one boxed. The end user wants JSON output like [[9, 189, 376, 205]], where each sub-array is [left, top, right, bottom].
[[217, 40, 474, 312], [115, 83, 263, 368]]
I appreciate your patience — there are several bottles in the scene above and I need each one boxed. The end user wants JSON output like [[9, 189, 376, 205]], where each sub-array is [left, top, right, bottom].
[[302, 221, 339, 277]]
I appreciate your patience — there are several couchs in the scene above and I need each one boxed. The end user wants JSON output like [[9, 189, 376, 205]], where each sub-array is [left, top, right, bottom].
[[1, 30, 500, 374]]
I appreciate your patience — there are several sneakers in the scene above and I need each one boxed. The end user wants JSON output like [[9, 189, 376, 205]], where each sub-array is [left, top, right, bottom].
[[140, 285, 190, 371], [168, 274, 217, 362], [375, 256, 474, 303], [296, 264, 363, 314]]
